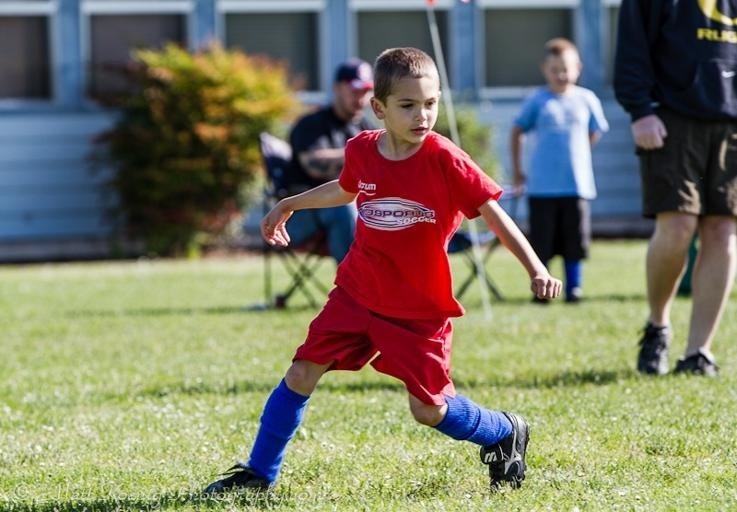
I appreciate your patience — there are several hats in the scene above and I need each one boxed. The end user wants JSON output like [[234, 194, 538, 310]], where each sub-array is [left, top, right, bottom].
[[334, 56, 376, 91]]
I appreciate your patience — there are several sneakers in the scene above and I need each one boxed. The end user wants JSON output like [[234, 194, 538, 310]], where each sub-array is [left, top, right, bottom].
[[636, 321, 672, 376], [532, 285, 584, 302], [202, 468, 275, 496], [675, 350, 719, 379], [479, 409, 530, 499]]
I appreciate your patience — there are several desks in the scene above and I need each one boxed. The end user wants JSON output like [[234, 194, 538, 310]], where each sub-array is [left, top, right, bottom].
[[285, 172, 529, 305]]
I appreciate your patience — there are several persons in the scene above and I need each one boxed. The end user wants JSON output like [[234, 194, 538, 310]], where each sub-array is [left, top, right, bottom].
[[277, 61, 379, 267], [511, 36, 608, 305], [201, 47, 563, 500], [615, 0, 736, 379]]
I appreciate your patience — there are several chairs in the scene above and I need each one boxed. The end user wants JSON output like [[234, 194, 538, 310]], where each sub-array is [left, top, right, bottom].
[[255, 130, 327, 308]]
